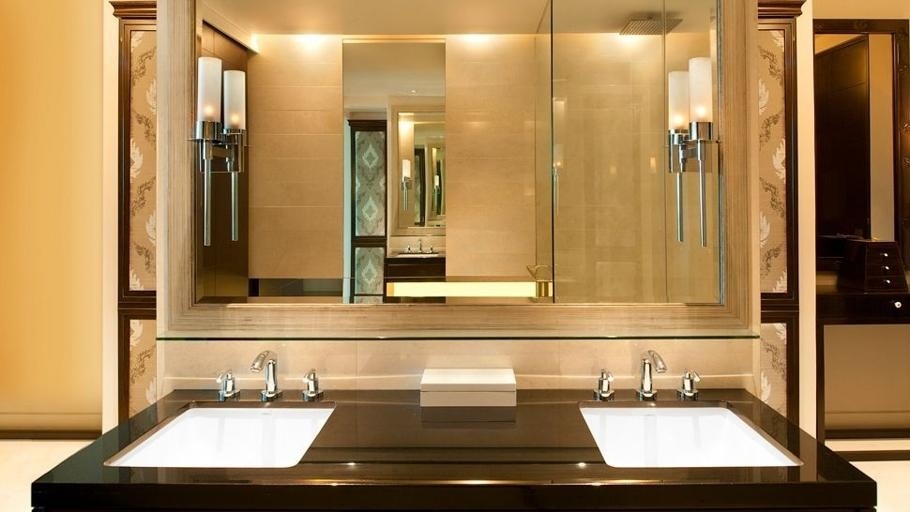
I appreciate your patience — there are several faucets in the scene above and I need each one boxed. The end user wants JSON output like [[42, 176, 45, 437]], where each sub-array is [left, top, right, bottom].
[[249, 348, 284, 402], [634, 349, 666, 397], [416, 238, 423, 251]]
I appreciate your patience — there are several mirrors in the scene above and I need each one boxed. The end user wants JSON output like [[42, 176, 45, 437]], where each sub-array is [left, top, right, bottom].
[[157, 0, 751, 331], [813, 19, 910, 272]]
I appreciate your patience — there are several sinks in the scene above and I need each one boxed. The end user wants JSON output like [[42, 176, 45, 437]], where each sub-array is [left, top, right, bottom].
[[394, 250, 441, 260], [574, 397, 803, 474], [101, 397, 340, 471]]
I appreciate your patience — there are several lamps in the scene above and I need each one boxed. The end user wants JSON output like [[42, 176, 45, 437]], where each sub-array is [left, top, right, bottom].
[[689, 57, 712, 249], [668, 70, 690, 242], [189, 57, 221, 246], [401, 159, 411, 211], [199, 70, 246, 242]]
